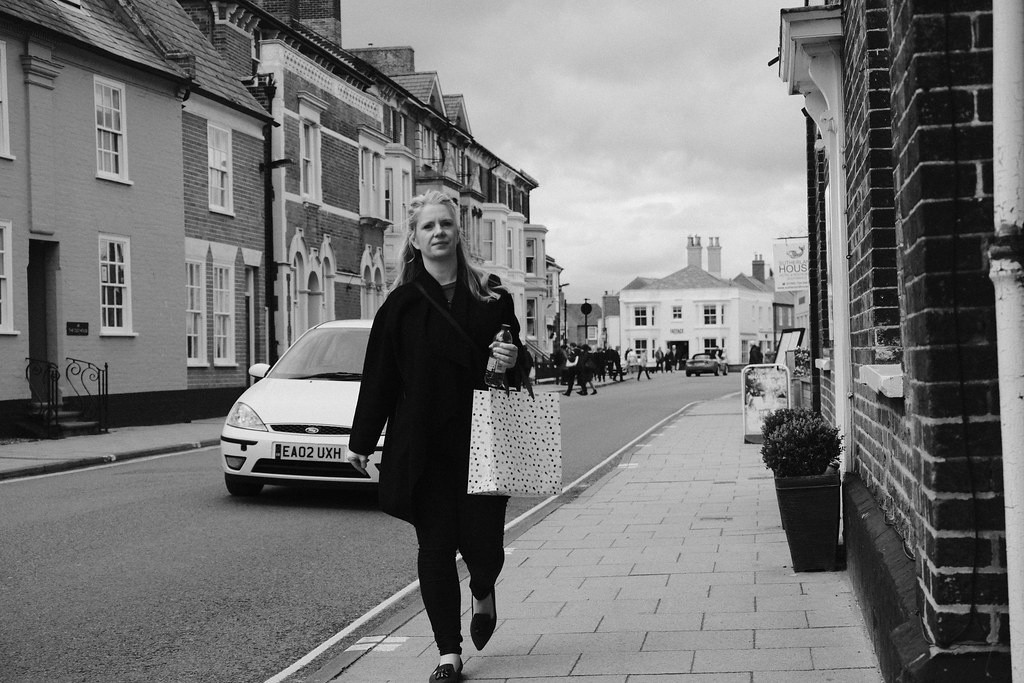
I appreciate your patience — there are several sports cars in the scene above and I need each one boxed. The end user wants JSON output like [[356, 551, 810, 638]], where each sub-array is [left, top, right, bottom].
[[685, 353, 729, 377]]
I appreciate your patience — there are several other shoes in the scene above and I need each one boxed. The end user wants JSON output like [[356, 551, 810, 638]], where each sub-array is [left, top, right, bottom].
[[469, 586, 497, 651], [620, 379, 625, 382], [576, 391, 582, 394], [429, 658, 463, 683], [580, 392, 588, 396], [591, 390, 597, 395], [562, 392, 570, 396], [613, 378, 617, 381]]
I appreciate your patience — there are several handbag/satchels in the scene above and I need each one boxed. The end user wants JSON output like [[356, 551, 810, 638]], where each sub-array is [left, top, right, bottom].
[[566, 351, 578, 367], [467, 354, 563, 496]]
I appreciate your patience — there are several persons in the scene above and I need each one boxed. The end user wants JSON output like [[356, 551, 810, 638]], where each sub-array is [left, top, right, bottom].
[[592, 345, 626, 383], [577, 344, 598, 395], [662, 349, 676, 373], [557, 344, 571, 385], [669, 345, 680, 372], [561, 343, 588, 396], [655, 347, 665, 373], [345, 189, 533, 683], [748, 340, 763, 369], [637, 350, 651, 381], [624, 347, 638, 374]]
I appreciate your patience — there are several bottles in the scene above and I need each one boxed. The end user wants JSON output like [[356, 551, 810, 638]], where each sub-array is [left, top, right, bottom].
[[485, 324, 512, 385]]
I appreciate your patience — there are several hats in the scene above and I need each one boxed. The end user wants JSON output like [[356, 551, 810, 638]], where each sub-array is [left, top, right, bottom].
[[581, 344, 592, 351]]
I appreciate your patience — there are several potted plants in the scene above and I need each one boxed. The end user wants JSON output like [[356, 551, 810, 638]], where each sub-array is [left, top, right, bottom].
[[763, 407, 841, 573]]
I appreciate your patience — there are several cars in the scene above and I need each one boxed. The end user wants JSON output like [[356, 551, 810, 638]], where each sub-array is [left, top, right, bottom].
[[221, 319, 390, 496]]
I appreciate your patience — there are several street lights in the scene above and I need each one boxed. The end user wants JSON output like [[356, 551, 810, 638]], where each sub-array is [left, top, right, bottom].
[[585, 299, 590, 345], [263, 158, 296, 367]]
